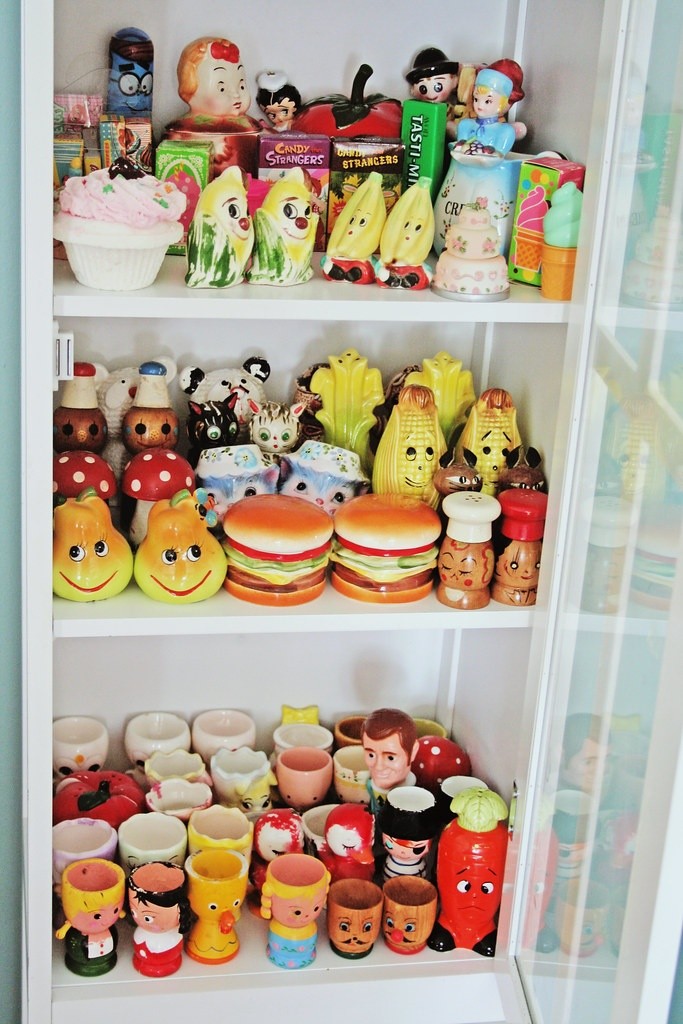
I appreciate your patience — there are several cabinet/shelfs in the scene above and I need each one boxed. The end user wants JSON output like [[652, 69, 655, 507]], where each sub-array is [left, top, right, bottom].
[[20, 0, 683, 1024]]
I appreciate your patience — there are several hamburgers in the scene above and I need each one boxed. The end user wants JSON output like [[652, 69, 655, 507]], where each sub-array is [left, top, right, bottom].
[[217, 494, 334, 607], [331, 493, 442, 603]]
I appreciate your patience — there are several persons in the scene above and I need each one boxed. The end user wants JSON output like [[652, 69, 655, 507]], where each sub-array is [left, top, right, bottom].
[[56, 859, 192, 966], [166, 37, 259, 134], [254, 71, 303, 134], [404, 47, 527, 156], [360, 708, 442, 886]]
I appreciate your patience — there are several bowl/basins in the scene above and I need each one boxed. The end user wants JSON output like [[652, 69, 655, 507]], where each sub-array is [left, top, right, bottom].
[[53, 213, 185, 292]]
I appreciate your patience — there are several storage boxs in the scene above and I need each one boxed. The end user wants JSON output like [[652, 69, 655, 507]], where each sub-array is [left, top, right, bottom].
[[401, 98, 447, 207], [85, 152, 101, 176], [508, 156, 585, 287], [125, 117, 153, 176], [326, 136, 406, 251], [53, 135, 84, 260], [156, 139, 214, 256], [54, 94, 103, 135], [98, 114, 125, 169], [257, 134, 331, 252]]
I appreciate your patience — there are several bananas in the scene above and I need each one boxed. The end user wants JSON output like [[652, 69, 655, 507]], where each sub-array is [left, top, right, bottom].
[[380, 175, 436, 265], [325, 172, 387, 260]]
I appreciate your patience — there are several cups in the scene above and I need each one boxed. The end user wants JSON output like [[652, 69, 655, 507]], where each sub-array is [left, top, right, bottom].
[[434, 141, 567, 260], [52, 710, 512, 978]]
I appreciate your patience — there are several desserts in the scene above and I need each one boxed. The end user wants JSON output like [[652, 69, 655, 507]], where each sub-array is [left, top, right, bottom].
[[53, 157, 186, 291]]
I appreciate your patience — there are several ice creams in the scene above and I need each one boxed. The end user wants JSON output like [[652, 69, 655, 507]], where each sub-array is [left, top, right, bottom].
[[514, 186, 549, 272], [539, 180, 585, 300]]
